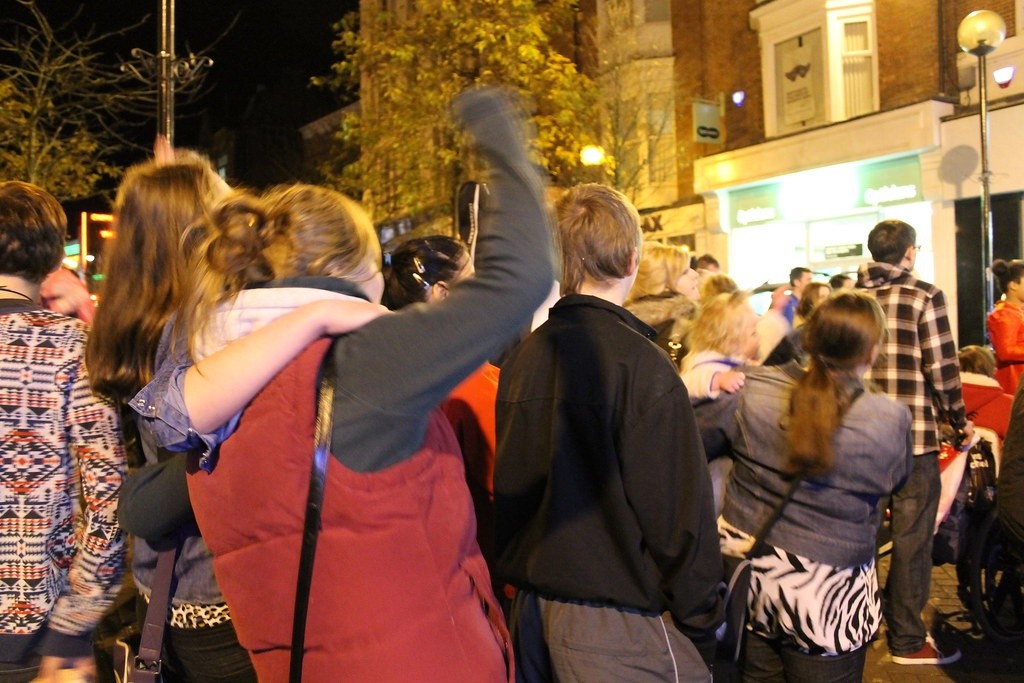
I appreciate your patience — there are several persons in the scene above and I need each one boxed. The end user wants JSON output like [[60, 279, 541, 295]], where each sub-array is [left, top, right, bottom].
[[0, 84, 1024, 683]]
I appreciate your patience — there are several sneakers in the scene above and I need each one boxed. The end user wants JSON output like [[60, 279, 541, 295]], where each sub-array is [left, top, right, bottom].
[[892, 633, 963, 664]]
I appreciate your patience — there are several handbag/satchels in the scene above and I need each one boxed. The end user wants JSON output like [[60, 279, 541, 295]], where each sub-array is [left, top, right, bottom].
[[716, 551, 752, 663], [114, 632, 163, 683]]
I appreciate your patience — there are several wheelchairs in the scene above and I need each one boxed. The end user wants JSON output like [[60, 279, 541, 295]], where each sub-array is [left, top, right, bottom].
[[929, 427, 1023, 650]]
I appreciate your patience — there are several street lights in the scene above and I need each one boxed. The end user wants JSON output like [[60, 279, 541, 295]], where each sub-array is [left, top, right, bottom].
[[956, 10, 1006, 345]]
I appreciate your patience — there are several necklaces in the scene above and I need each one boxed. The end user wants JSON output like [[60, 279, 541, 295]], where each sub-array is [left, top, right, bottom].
[[0, 288, 32, 300]]
